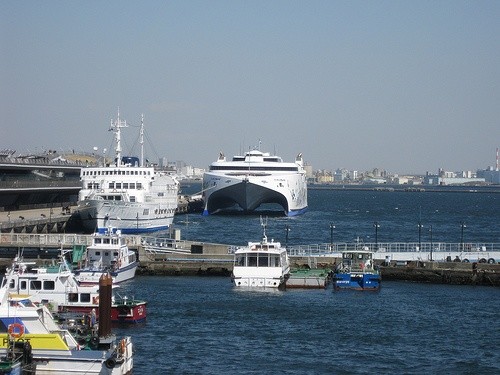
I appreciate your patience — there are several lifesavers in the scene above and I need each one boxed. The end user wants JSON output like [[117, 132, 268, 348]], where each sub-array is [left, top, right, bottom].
[[105, 358, 116, 369], [95, 296, 99, 304], [121, 341, 125, 354], [9, 323, 24, 338], [480, 258, 486, 263], [463, 258, 469, 263], [488, 258, 495, 264]]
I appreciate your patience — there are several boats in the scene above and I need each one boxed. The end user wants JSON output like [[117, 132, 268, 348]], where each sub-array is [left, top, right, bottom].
[[57, 228, 140, 285], [331, 251, 380, 289], [202, 138, 310, 217], [231, 215, 291, 287], [76, 105, 186, 238], [0, 270, 136, 375], [0, 242, 148, 324]]
